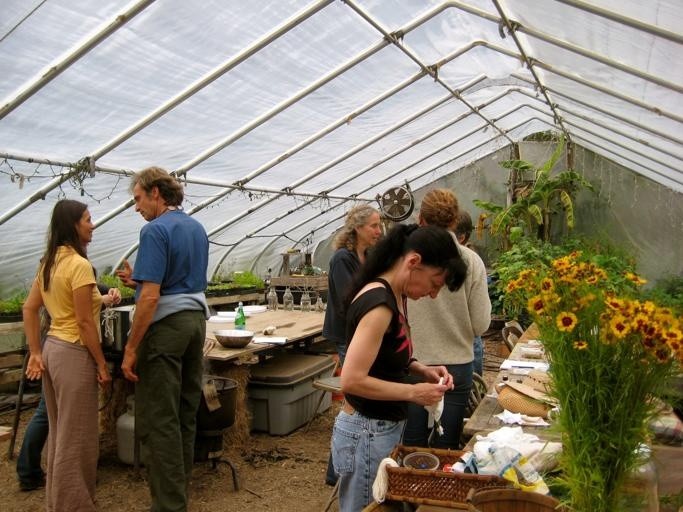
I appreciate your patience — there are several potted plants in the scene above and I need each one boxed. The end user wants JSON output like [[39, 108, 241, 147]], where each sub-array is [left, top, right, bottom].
[[96, 270, 136, 312], [0, 273, 33, 322], [205, 264, 267, 299]]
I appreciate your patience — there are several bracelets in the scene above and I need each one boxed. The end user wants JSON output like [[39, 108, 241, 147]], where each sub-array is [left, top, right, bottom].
[[408, 358, 417, 367]]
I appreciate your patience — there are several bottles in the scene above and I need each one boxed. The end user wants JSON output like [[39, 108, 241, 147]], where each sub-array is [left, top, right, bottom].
[[278, 256, 292, 278], [303, 253, 314, 276], [266, 287, 324, 314], [233, 301, 247, 330]]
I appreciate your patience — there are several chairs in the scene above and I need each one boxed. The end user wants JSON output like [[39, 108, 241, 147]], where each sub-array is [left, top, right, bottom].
[[323, 471, 407, 512], [502, 321, 524, 353], [304, 354, 344, 434]]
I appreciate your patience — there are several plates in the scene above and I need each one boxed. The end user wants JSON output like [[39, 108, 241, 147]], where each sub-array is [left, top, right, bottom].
[[211, 306, 265, 324]]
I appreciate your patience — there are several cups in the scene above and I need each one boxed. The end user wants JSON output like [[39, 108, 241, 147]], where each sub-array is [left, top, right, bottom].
[[402, 451, 441, 472]]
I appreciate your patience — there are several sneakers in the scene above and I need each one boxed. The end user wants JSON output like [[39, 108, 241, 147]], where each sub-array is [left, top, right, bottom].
[[19, 475, 47, 490]]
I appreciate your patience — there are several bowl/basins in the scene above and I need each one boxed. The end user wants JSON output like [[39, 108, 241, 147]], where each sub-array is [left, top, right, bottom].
[[214, 330, 252, 347]]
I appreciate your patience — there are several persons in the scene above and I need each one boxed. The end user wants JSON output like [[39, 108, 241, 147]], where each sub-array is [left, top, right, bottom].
[[328, 222, 470, 511], [15, 282, 124, 493], [113, 165, 211, 511], [20, 199, 114, 511], [397, 187, 491, 452], [320, 204, 383, 487], [449, 209, 493, 376]]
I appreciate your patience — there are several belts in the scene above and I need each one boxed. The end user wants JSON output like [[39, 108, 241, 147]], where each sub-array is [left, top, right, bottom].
[[341, 399, 356, 415]]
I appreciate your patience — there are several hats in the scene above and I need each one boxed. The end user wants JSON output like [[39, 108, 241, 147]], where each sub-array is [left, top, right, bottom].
[[506, 368, 561, 409]]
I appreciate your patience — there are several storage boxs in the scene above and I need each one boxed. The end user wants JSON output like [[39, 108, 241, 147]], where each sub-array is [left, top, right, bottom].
[[247, 351, 336, 436]]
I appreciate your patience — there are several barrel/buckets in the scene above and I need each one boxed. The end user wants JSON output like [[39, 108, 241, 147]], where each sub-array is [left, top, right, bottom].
[[196, 375, 238, 426], [465, 485, 570, 511]]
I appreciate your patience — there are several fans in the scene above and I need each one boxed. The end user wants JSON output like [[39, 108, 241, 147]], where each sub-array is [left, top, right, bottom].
[[381, 186, 414, 221]]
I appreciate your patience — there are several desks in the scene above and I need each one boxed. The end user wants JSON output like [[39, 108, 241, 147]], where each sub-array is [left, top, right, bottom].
[[416, 431, 682, 512], [203, 303, 328, 481], [462, 320, 683, 435]]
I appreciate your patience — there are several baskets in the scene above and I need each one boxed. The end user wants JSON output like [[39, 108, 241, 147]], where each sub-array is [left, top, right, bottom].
[[385, 444, 513, 508]]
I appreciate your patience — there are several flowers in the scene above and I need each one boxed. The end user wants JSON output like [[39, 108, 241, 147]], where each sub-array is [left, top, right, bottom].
[[491, 248, 683, 512]]
